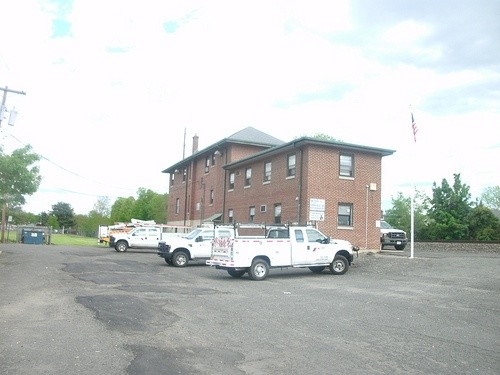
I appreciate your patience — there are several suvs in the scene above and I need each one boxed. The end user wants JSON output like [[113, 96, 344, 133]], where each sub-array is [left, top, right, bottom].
[[380, 220, 408, 251]]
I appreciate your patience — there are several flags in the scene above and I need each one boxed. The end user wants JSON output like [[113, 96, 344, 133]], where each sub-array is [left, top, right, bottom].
[[411, 110, 417, 142]]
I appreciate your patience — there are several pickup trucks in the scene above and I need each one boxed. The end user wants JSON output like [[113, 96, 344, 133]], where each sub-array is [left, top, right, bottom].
[[108, 224, 355, 280]]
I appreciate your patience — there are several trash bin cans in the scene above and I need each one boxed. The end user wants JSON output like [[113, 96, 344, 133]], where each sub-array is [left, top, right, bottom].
[[20, 225, 46, 245]]
